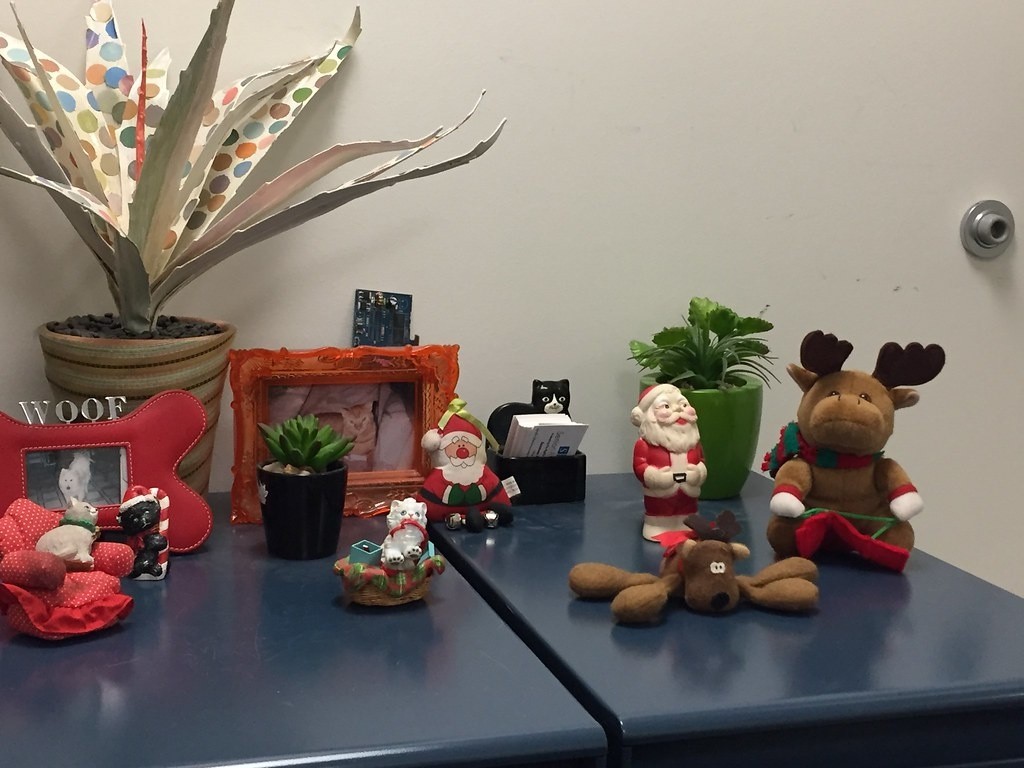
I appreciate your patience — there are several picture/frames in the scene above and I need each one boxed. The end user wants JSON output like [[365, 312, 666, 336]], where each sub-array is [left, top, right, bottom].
[[24, 444, 130, 507], [227, 342, 459, 527]]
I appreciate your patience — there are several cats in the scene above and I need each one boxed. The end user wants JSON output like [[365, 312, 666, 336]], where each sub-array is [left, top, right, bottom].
[[380, 497, 427, 570], [315, 400, 376, 456], [116, 501, 168, 578], [35, 496, 99, 572], [485, 378, 571, 449]]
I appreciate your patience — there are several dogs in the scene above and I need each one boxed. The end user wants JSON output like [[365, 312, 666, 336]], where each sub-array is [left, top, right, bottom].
[[58, 448, 95, 503]]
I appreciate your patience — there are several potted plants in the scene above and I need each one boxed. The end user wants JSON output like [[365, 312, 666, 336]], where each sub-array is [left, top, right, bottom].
[[252, 413, 357, 562], [0, 0, 506, 508], [625, 296, 783, 501]]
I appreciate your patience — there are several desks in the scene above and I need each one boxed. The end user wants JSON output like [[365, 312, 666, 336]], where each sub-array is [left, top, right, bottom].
[[0, 488, 614, 768], [433, 464, 1023, 766]]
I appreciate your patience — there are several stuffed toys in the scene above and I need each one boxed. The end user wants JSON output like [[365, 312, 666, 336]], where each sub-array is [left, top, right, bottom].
[[530, 379, 572, 420], [760, 329, 947, 571], [567, 511, 821, 618], [415, 398, 518, 530]]
